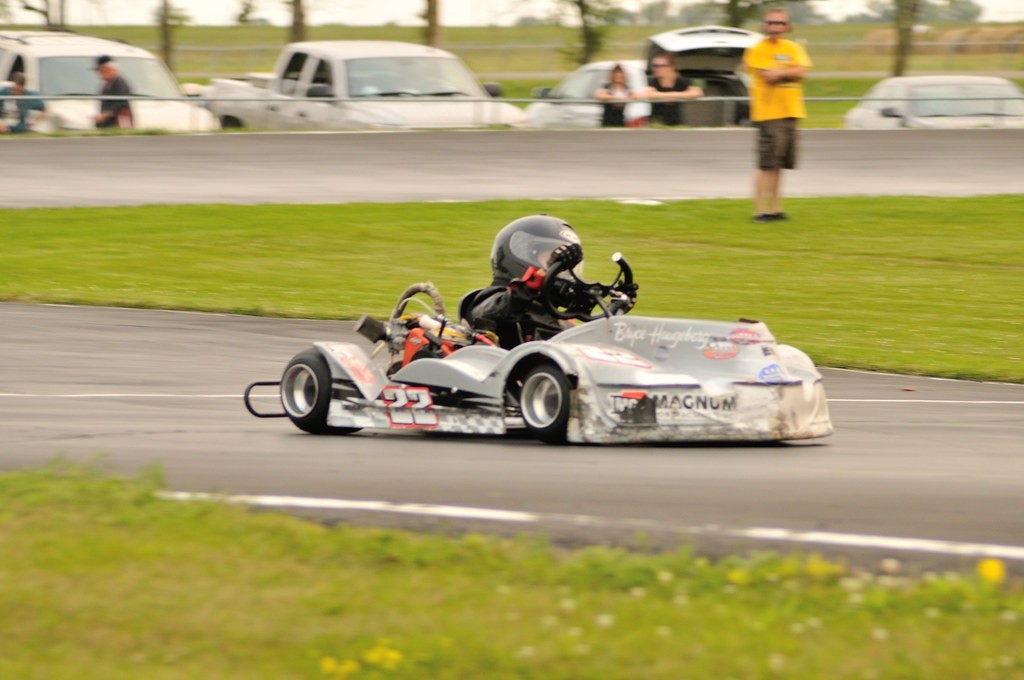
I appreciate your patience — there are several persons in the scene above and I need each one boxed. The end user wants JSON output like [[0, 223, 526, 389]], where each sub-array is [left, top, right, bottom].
[[467, 215, 637, 350], [595, 52, 749, 127], [744, 9, 811, 221], [94, 56, 132, 128], [0, 73, 45, 133]]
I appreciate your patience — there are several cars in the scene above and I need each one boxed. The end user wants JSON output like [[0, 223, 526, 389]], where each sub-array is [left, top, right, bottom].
[[843, 74, 1023, 130], [529, 26, 772, 127]]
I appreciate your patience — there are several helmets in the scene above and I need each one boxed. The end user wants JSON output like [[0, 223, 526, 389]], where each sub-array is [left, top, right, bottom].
[[487, 213, 585, 303]]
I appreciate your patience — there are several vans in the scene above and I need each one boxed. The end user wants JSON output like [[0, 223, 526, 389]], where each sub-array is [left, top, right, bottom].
[[1, 28, 224, 134]]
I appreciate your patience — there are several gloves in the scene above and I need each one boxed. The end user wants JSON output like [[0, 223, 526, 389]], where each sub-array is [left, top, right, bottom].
[[609, 280, 638, 313], [546, 243, 582, 268]]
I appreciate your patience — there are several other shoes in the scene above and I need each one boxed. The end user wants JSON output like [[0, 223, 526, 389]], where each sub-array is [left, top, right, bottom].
[[751, 212, 789, 225]]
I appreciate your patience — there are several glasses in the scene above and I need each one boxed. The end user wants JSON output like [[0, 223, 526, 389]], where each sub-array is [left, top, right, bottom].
[[764, 20, 788, 26]]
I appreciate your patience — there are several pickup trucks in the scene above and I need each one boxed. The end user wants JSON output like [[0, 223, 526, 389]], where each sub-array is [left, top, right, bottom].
[[201, 39, 532, 131]]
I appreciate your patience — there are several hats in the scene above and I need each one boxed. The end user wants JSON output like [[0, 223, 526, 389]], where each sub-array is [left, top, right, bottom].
[[12, 72, 26, 86], [88, 55, 112, 69]]
